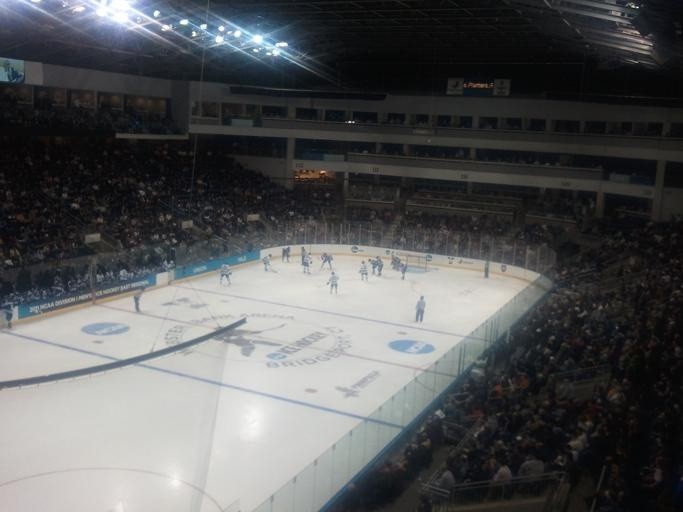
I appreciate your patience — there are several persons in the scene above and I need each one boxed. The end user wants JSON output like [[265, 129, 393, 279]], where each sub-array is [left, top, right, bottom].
[[0, 83, 145, 313], [145, 98, 337, 276], [218, 261, 232, 287], [325, 271, 339, 295], [336, 106, 683, 512]]
